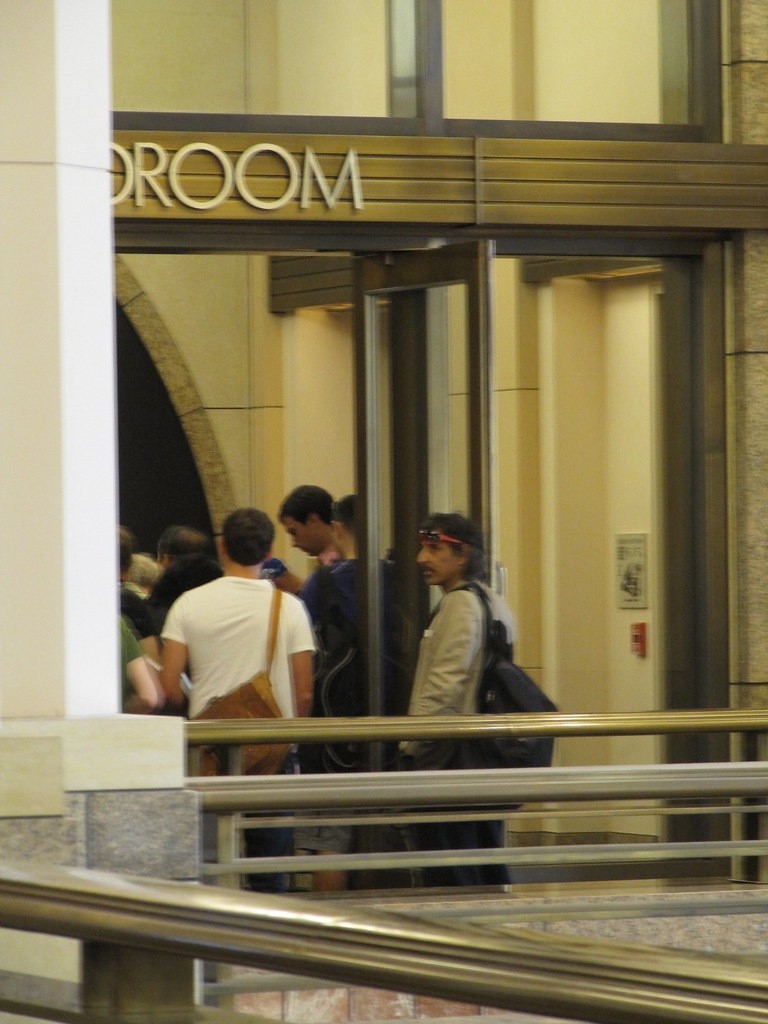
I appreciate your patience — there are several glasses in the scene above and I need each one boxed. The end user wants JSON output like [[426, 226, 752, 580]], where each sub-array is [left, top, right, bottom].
[[419, 531, 463, 545]]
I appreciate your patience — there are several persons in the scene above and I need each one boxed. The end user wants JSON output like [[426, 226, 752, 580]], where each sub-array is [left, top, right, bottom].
[[122, 479, 432, 713], [307, 493, 416, 888], [157, 509, 318, 894], [397, 511, 515, 891]]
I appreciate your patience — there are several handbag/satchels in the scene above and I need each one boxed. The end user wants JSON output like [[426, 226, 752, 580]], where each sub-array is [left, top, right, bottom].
[[427, 582, 557, 814], [188, 670, 291, 775]]
